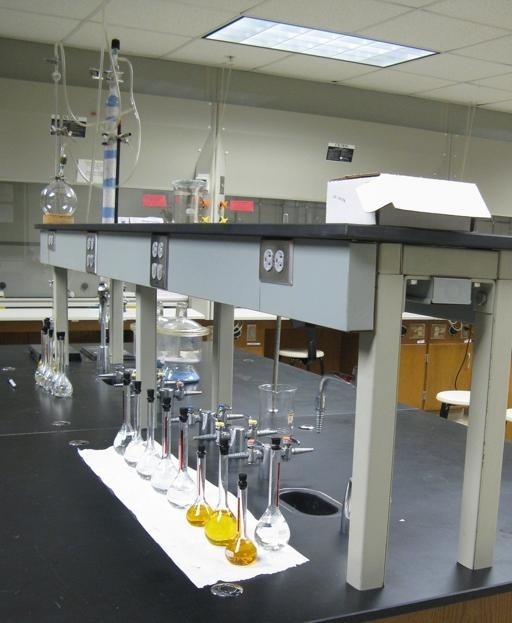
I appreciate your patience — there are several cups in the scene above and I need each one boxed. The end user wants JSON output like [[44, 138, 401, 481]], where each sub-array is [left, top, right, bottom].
[[172, 179, 206, 223], [259, 384, 298, 435]]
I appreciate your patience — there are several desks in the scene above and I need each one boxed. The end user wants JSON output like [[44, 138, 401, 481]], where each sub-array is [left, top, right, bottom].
[[0, 341, 512, 622]]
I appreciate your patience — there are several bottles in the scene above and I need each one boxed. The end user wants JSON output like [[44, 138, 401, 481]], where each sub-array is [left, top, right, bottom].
[[37, 329, 48, 387], [255, 438, 290, 549], [38, 321, 49, 369], [187, 445, 213, 525], [205, 439, 242, 545], [40, 176, 75, 215], [54, 332, 73, 398], [113, 371, 136, 455], [52, 332, 62, 394], [43, 330, 54, 390], [125, 381, 145, 468], [226, 474, 256, 565], [136, 389, 162, 477], [167, 407, 199, 509], [151, 397, 178, 495]]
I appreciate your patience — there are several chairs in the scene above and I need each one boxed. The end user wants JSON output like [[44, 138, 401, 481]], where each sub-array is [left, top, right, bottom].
[[278, 317, 326, 376], [436, 389, 471, 418]]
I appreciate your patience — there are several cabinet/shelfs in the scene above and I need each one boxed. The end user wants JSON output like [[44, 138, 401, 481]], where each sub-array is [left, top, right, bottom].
[[398, 312, 473, 412]]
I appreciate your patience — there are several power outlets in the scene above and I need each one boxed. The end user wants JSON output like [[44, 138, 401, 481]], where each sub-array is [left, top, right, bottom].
[[259, 238, 293, 285], [86, 233, 97, 273], [48, 230, 56, 248], [151, 234, 168, 289]]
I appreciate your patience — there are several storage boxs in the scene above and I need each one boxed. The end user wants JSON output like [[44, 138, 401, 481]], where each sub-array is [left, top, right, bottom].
[[326, 173, 493, 231]]
